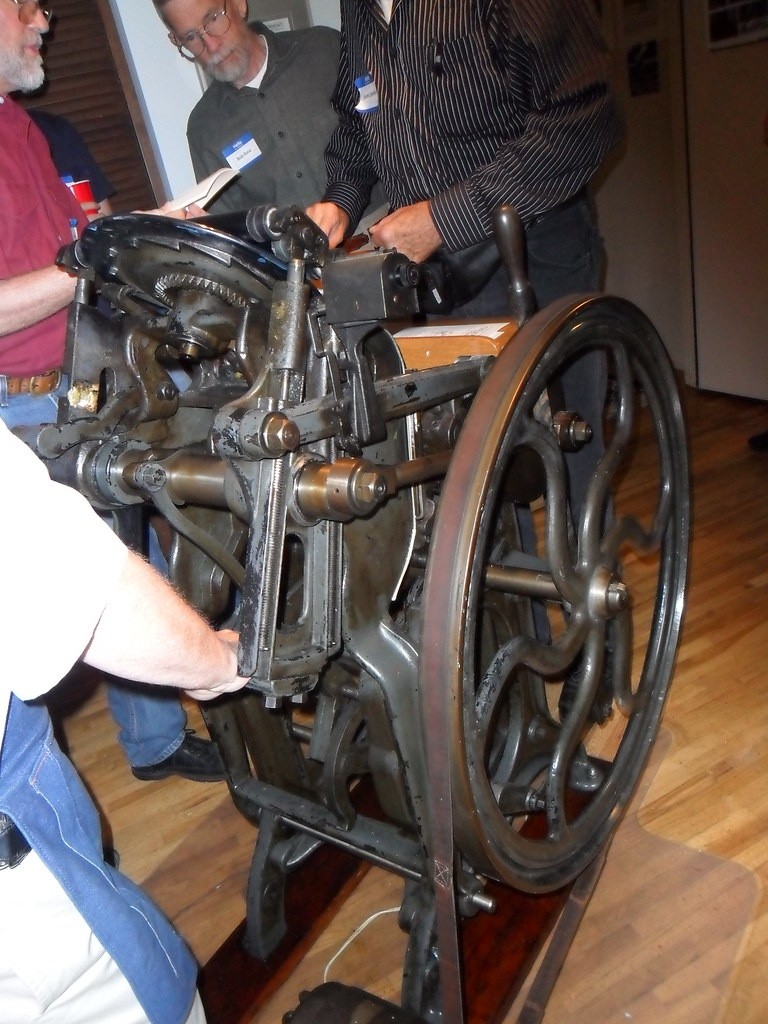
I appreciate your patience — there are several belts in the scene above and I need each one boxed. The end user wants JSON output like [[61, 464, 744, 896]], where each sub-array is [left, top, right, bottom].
[[6, 370, 62, 397], [0, 815, 32, 868], [522, 186, 587, 234], [341, 208, 394, 253]]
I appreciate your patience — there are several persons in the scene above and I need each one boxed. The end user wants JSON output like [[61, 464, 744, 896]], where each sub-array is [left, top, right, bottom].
[[0, 0, 250, 1024], [153, 0, 624, 722]]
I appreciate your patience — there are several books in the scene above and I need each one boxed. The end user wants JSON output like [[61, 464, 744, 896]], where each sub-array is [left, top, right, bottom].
[[159, 168, 240, 211]]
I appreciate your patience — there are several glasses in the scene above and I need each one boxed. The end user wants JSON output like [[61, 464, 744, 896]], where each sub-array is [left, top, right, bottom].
[[11, 0, 53, 25], [171, 0, 230, 62]]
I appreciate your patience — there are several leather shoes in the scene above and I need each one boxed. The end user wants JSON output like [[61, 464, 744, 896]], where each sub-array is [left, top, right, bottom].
[[131, 728, 226, 782]]
